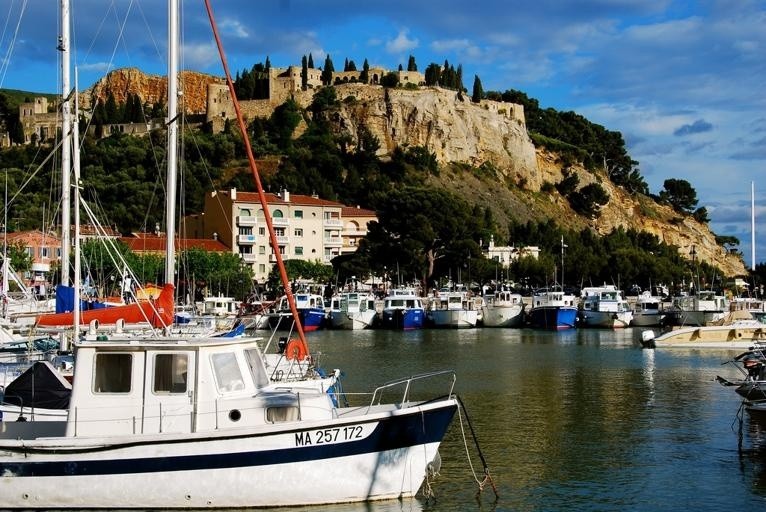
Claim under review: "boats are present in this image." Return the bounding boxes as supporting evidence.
[0,316,465,511]
[1,0,766,415]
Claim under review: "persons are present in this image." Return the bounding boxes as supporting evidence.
[123,270,137,305]
